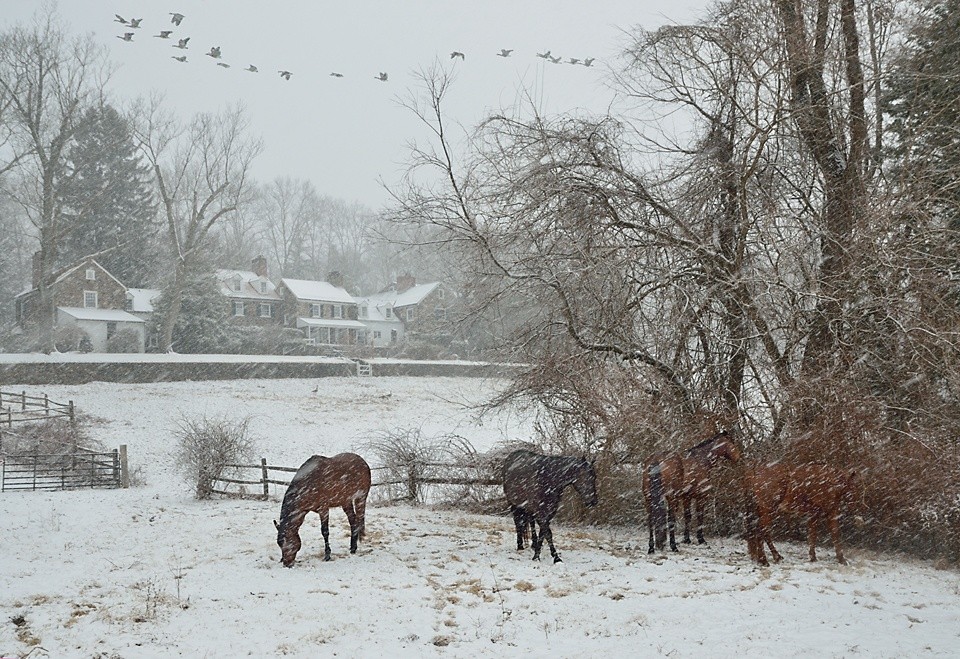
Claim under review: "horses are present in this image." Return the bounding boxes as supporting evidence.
[642,430,857,570]
[503,449,596,563]
[273,452,372,568]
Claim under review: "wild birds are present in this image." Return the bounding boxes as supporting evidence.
[113,9,597,82]
